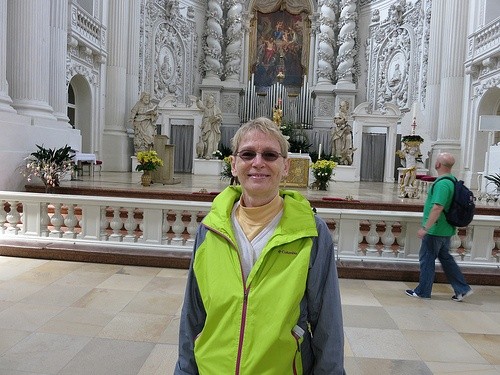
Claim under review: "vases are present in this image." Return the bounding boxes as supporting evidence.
[318,171,332,191]
[229,177,240,186]
[141,171,153,186]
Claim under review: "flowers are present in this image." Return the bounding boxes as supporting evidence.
[135,149,164,173]
[16,158,59,190]
[309,158,338,190]
[217,155,236,180]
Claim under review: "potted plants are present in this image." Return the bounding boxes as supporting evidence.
[401,133,425,146]
[30,143,77,186]
[484,172,500,194]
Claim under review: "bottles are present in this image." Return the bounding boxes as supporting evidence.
[290,324,305,342]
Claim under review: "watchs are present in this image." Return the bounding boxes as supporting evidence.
[423,226,428,231]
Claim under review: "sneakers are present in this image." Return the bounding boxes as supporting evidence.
[406,289,430,299]
[452,289,473,302]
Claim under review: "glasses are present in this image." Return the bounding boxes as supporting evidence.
[236,150,285,161]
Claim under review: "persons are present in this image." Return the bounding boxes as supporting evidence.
[129,91,158,151]
[160,23,169,47]
[196,94,223,160]
[396,29,406,48]
[168,26,177,48]
[389,63,401,86]
[196,136,207,159]
[329,111,352,165]
[402,146,418,188]
[170,0,185,20]
[273,104,283,128]
[404,151,473,302]
[390,5,399,26]
[162,55,172,79]
[383,31,397,56]
[173,117,346,375]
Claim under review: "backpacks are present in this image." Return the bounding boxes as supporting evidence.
[433,177,476,227]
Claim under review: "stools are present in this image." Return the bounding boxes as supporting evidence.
[83,160,103,176]
[400,174,428,192]
[420,176,437,196]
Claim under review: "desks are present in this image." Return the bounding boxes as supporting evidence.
[71,152,97,178]
[279,157,311,188]
[397,167,429,184]
